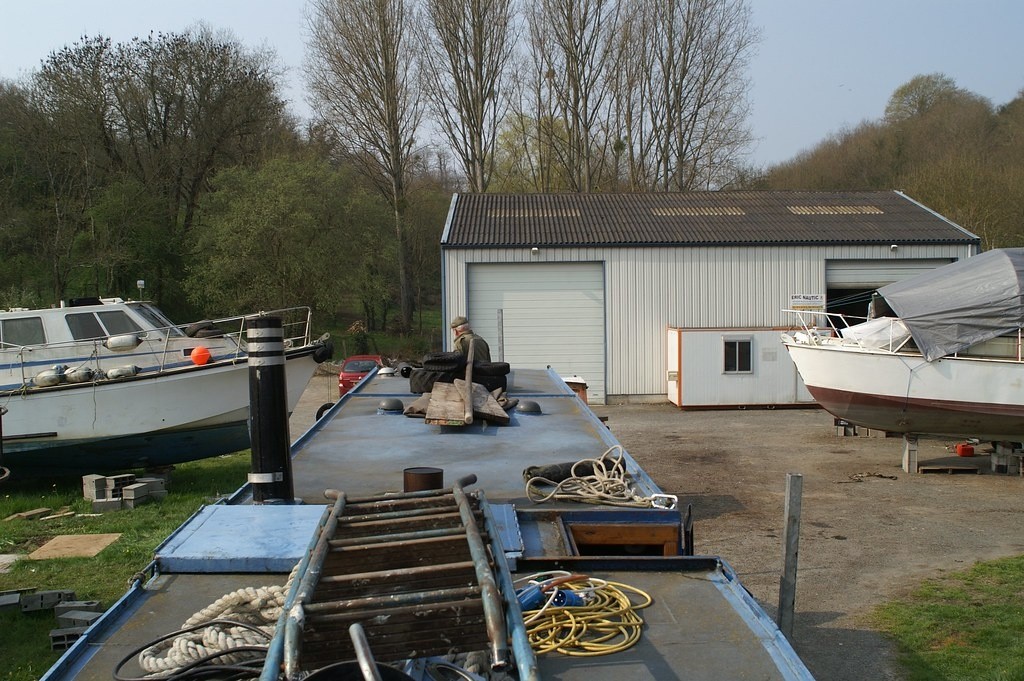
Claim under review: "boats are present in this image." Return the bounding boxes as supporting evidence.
[0,296,330,479]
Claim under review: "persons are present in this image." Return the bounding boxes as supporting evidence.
[450,316,492,363]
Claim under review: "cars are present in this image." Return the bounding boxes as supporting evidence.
[339,354,389,398]
[779,247,1024,447]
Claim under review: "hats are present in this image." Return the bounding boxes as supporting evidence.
[451,316,468,328]
